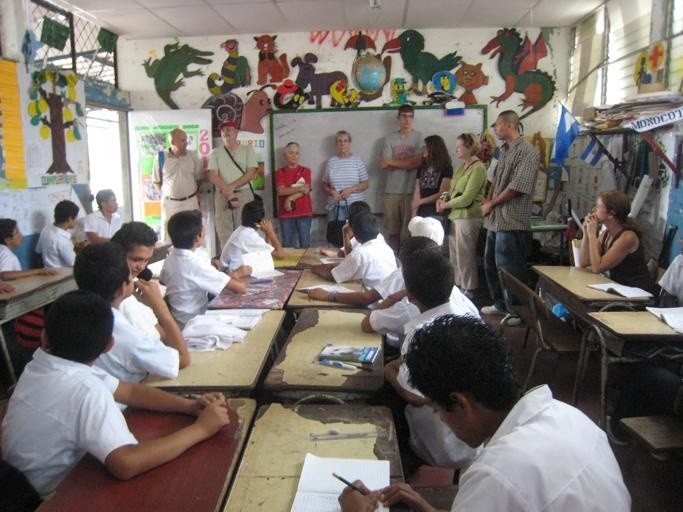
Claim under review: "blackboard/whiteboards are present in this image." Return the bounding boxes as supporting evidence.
[269,105,488,219]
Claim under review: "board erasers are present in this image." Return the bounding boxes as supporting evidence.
[447,108,464,115]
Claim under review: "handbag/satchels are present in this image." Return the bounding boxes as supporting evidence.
[326,197,351,244]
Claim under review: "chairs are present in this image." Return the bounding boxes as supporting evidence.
[498,266,600,397]
[650,265,667,307]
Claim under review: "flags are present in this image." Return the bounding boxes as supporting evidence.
[549,106,580,167]
[580,135,604,167]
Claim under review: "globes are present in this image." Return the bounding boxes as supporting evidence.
[351,56,387,92]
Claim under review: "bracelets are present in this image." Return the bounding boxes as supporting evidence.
[327,290,337,303]
[351,186,356,193]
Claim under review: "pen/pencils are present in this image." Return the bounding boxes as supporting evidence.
[332,472,366,496]
[346,363,372,372]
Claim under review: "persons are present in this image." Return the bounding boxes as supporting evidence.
[0,289,229,511]
[158,210,246,332]
[151,128,202,242]
[578,189,659,310]
[657,232,682,308]
[409,135,452,237]
[310,210,398,308]
[605,362,682,462]
[73,240,190,417]
[308,215,444,356]
[341,204,385,258]
[205,121,259,252]
[375,104,424,239]
[321,130,369,246]
[33,199,91,269]
[0,283,16,296]
[479,109,539,325]
[219,200,284,275]
[380,247,485,470]
[108,220,169,347]
[274,142,312,248]
[317,200,371,257]
[0,218,57,281]
[435,132,487,299]
[337,313,631,512]
[360,236,481,410]
[191,208,251,280]
[83,189,124,244]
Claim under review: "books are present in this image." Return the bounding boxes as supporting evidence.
[587,282,653,299]
[296,283,355,295]
[645,306,683,334]
[205,306,270,330]
[319,344,378,365]
[319,256,344,265]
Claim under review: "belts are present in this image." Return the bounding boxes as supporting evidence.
[166,193,199,202]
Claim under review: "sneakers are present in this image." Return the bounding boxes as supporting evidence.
[482,304,506,315]
[500,311,522,325]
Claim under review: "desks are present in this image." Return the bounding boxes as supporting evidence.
[530,267,651,384]
[527,215,571,266]
[1,266,79,384]
[206,247,368,334]
[572,311,683,431]
[262,306,386,403]
[223,403,410,512]
[147,242,173,266]
[619,413,683,511]
[139,307,286,399]
[35,397,258,511]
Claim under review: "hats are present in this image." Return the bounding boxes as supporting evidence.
[219,119,236,130]
